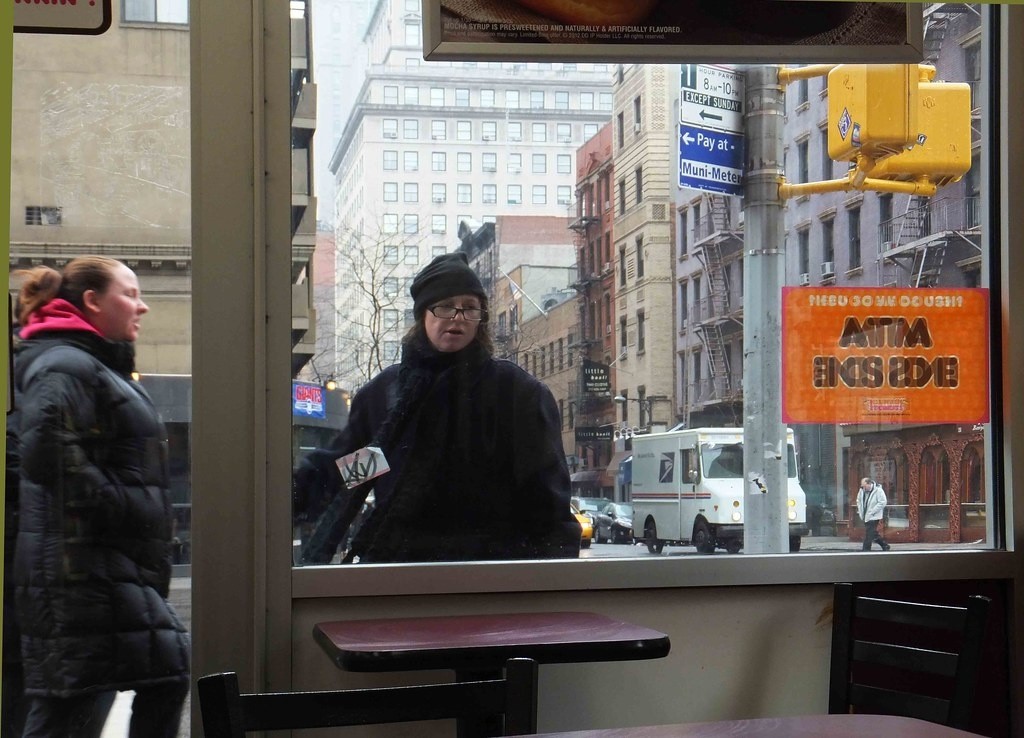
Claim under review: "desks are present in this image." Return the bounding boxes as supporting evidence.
[313,610,671,738]
[493,715,989,738]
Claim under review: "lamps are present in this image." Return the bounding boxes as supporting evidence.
[613,395,674,406]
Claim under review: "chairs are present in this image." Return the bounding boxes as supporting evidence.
[197,656,540,738]
[822,582,991,730]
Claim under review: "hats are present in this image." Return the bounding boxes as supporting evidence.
[409,251,484,319]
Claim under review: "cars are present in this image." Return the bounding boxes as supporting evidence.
[571,494,633,550]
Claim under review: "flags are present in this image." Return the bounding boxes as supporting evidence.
[509,279,524,301]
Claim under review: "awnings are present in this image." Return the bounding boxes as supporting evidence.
[619,455,632,485]
[569,471,599,482]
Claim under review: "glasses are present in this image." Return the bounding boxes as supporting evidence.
[426,305,488,321]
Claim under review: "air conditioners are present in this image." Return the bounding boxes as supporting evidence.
[821,262,834,277]
[881,242,891,253]
[800,272,810,286]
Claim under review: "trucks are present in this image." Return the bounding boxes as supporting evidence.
[632,421,806,555]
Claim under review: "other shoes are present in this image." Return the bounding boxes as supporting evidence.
[883,545,890,551]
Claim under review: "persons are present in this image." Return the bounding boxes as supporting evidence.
[10,256,191,738]
[296,252,582,566]
[856,477,890,552]
